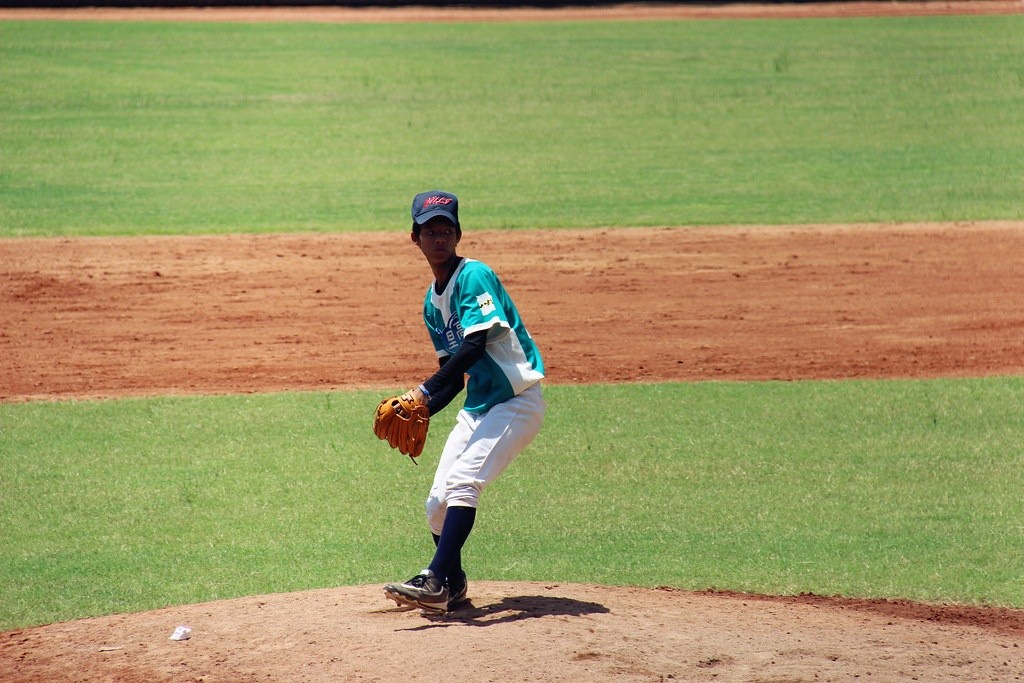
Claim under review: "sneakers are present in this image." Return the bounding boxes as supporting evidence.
[445,572,466,606]
[382,567,449,618]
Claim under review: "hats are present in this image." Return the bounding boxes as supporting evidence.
[411,191,458,225]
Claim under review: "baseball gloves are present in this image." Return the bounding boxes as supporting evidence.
[372,388,430,458]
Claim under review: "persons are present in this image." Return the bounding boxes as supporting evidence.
[372,191,544,616]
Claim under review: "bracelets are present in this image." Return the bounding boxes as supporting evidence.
[419,384,429,396]
